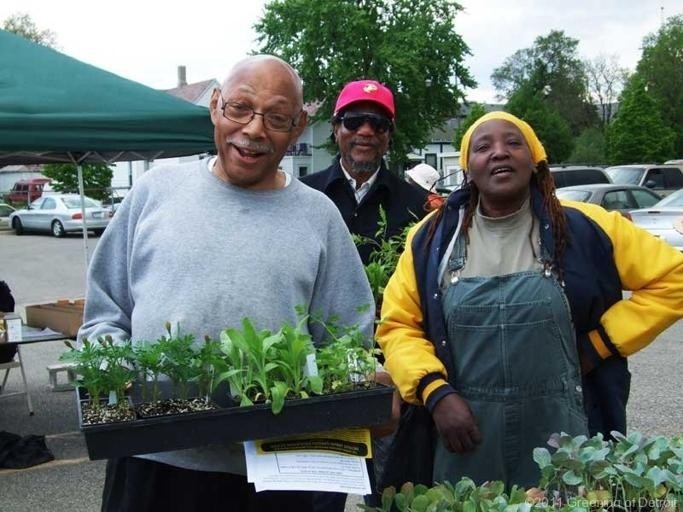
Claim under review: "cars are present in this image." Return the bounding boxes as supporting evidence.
[430,158,683,255]
[0,177,133,238]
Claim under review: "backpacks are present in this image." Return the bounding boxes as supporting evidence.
[422,192,445,212]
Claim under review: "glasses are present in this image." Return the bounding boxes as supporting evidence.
[216,87,304,134]
[336,110,393,134]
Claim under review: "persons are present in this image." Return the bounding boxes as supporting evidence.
[404,163,445,209]
[77,54,376,512]
[374,113,683,497]
[296,80,446,511]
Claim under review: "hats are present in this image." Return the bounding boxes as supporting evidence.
[332,79,396,120]
[403,162,440,194]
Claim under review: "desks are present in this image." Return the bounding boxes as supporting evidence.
[0,324,69,346]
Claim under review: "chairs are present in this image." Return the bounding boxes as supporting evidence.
[0,344,34,415]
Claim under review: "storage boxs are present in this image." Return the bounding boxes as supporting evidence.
[25,299,84,338]
